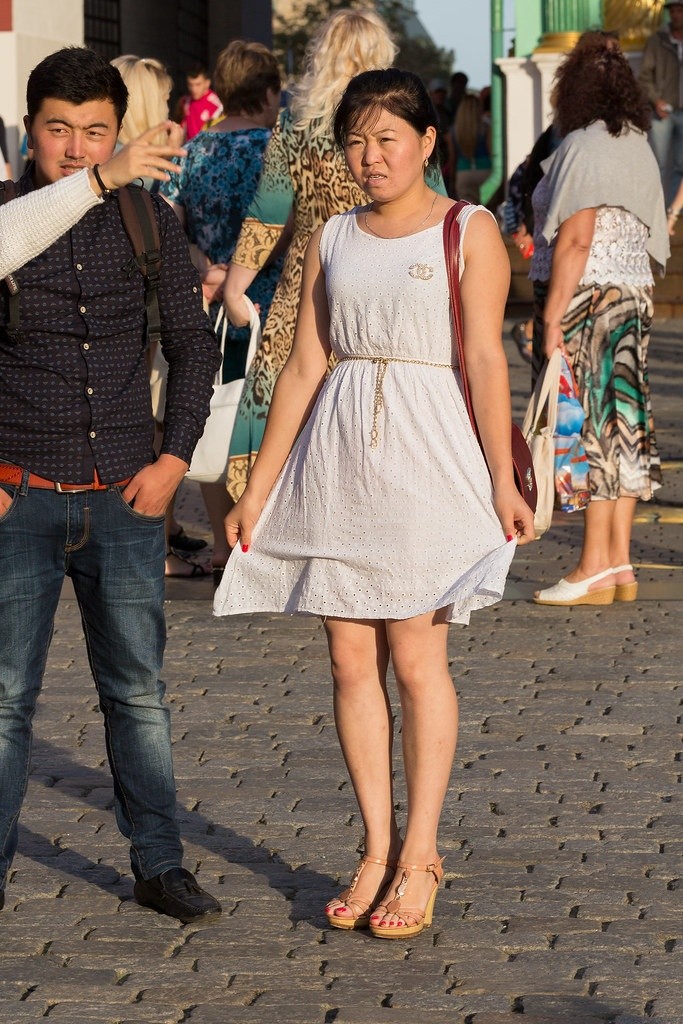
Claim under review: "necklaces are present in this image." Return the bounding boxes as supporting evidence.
[365,195,438,240]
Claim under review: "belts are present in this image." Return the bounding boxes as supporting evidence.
[0,463,153,494]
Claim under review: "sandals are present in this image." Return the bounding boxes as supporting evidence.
[164,550,211,577]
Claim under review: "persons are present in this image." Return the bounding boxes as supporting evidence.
[508,0,683,606]
[1,12,448,924]
[214,69,537,938]
[429,72,491,202]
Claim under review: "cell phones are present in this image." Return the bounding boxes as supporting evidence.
[512,233,534,258]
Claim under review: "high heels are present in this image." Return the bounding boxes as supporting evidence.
[211,548,234,585]
[527,569,615,607]
[371,851,447,940]
[326,840,404,930]
[613,563,638,602]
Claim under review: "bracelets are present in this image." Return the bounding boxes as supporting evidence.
[667,214,678,221]
[667,208,680,216]
[93,164,111,195]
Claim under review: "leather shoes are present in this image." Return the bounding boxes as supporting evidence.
[132,868,222,921]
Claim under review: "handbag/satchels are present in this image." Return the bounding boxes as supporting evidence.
[518,349,565,540]
[511,420,538,517]
[183,293,262,484]
[555,357,590,514]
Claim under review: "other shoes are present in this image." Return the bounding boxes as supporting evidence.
[168,528,208,552]
